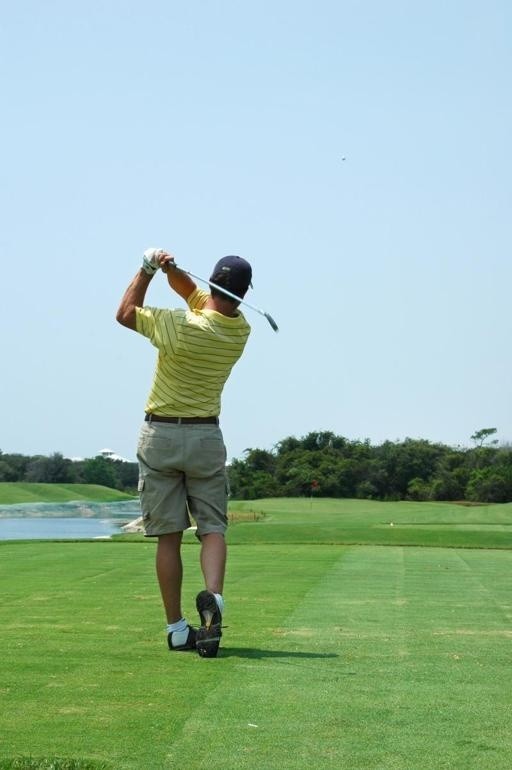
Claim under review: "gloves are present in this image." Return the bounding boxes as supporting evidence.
[140,247,168,275]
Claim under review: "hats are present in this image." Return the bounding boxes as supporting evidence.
[211,254,253,291]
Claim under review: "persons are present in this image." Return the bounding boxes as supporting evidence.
[114,246,257,660]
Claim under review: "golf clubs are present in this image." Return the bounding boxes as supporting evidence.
[166,262,279,333]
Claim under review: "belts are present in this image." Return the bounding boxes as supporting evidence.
[143,414,220,425]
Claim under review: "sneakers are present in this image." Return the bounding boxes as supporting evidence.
[166,624,198,651]
[194,591,225,660]
